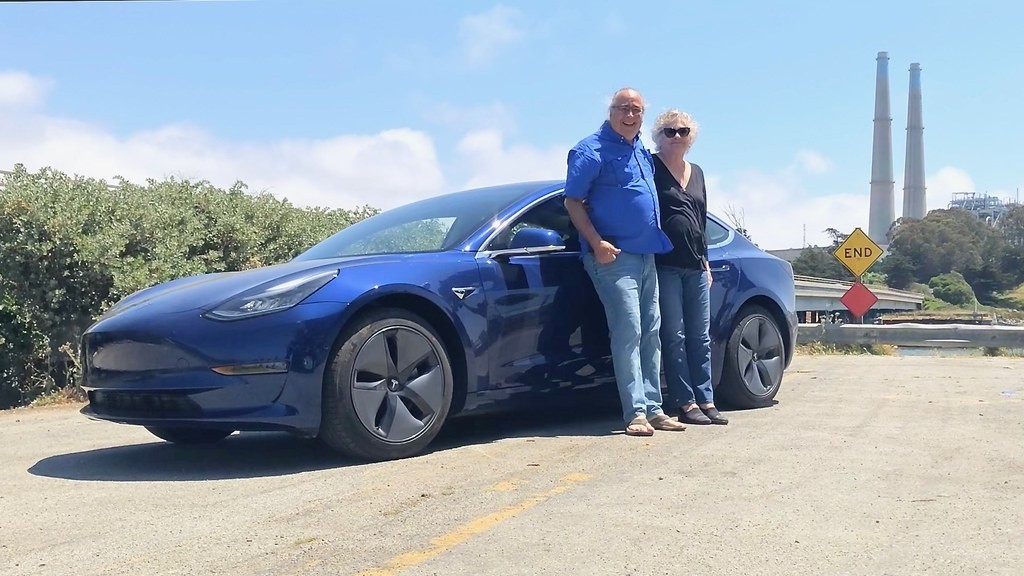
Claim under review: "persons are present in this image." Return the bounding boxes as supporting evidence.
[563,87,688,438]
[647,108,729,427]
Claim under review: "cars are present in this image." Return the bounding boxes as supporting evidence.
[79,180,798,461]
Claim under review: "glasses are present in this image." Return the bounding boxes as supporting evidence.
[660,128,690,138]
[612,106,643,115]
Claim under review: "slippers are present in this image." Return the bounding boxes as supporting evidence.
[626,415,686,435]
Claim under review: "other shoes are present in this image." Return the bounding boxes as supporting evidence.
[678,404,728,425]
